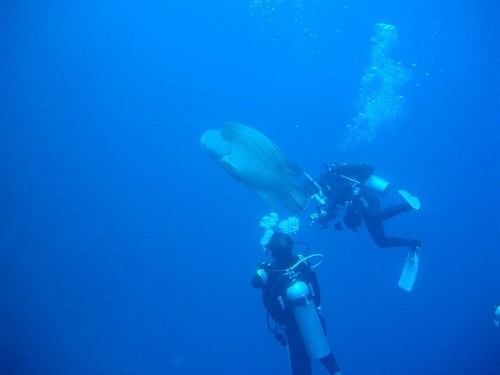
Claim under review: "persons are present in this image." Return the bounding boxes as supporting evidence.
[304,162,422,292]
[262,232,340,375]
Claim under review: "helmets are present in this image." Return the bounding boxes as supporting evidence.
[270,232,293,261]
[304,177,317,192]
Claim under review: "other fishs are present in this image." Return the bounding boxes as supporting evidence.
[199,122,321,214]
